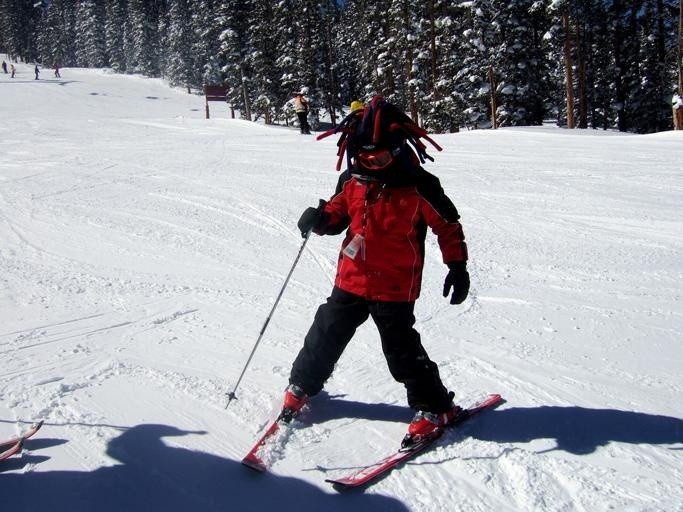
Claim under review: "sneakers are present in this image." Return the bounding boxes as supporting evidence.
[284,384,309,410]
[408,404,456,437]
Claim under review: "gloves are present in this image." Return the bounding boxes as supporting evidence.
[297,207,320,234]
[443,262,470,304]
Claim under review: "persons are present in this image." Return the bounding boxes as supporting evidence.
[295,86,312,135]
[11,65,16,77]
[34,64,40,79]
[54,64,61,78]
[2,60,8,74]
[284,96,470,436]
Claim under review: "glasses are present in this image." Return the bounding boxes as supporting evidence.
[357,148,400,170]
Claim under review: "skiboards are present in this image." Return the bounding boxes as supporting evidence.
[239,393,502,486]
[0,420,43,460]
